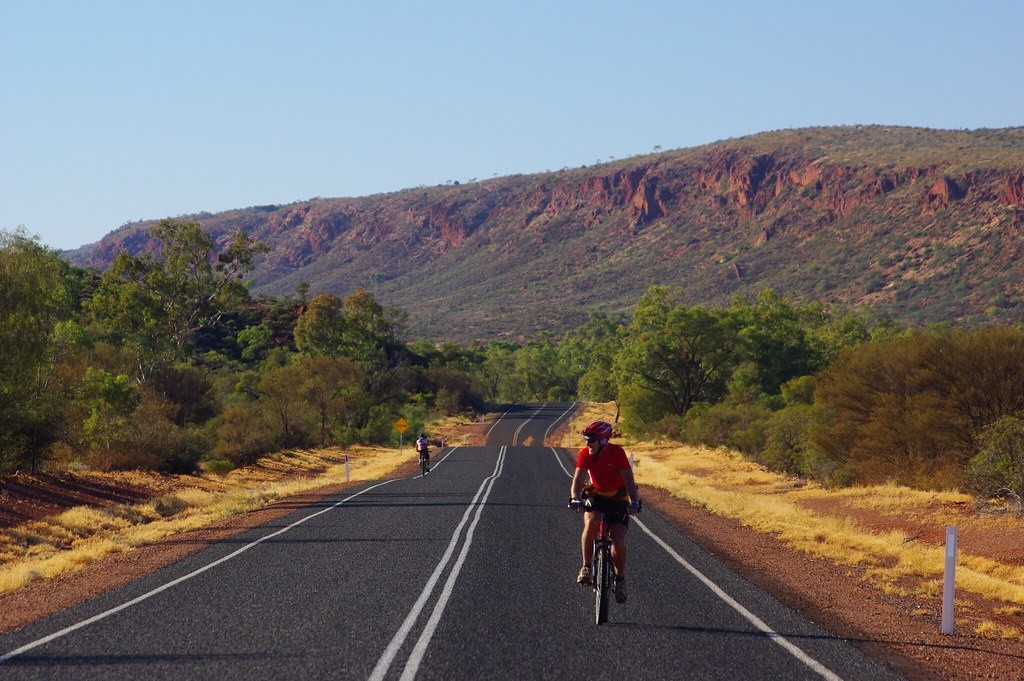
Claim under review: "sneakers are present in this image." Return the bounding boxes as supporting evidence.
[576,566,592,583]
[613,576,628,603]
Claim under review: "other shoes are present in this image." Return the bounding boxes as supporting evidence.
[426,468,430,472]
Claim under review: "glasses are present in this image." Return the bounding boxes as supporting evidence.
[585,438,599,443]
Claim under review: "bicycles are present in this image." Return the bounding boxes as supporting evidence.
[419,450,432,477]
[568,498,642,625]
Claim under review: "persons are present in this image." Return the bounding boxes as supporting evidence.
[568,421,639,603]
[415,433,430,472]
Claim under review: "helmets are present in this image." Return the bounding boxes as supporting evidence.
[419,434,427,438]
[581,422,613,438]
